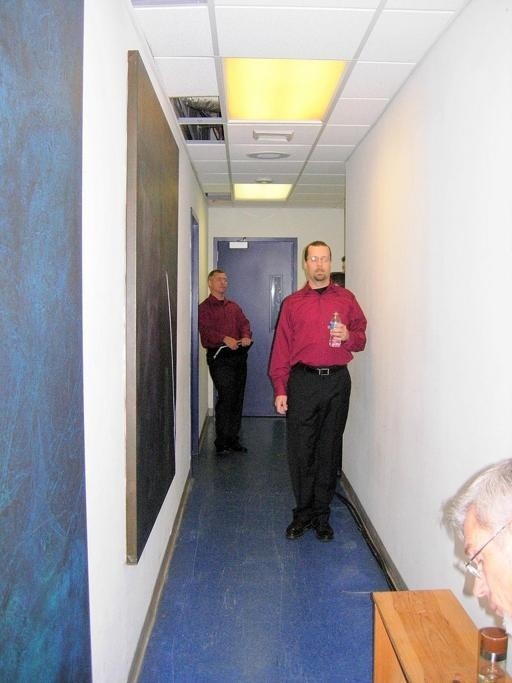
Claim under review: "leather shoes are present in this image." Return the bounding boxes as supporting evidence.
[213,436,248,456]
[286,515,333,541]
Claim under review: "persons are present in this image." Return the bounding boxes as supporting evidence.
[268,241,368,541]
[449,458,512,619]
[198,270,251,454]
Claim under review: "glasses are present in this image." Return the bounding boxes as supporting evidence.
[465,522,509,578]
[306,256,332,263]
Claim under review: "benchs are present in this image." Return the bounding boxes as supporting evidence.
[368,586,508,683]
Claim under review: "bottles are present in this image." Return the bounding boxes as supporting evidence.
[476,627,509,682]
[328,311,342,348]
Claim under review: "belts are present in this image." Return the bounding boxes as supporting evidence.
[297,360,346,376]
